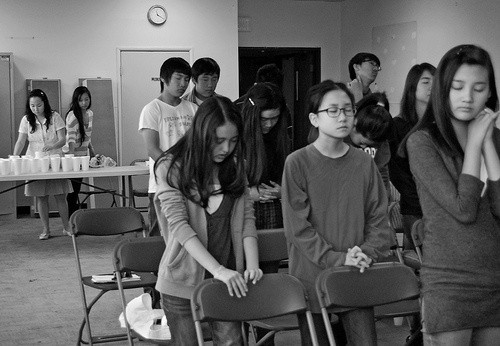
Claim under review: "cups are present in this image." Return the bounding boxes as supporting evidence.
[81,156,90,170]
[0,152,50,176]
[50,154,60,172]
[62,154,81,171]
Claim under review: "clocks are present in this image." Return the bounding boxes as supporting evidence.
[147,5,168,25]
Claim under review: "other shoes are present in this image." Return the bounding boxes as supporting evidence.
[63,229,72,236]
[39,233,50,240]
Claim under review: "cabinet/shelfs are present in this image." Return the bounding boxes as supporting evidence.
[0,52,17,222]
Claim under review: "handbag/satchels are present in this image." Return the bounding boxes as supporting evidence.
[119,293,171,340]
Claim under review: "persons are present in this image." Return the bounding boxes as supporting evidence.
[233,56,294,346]
[65,86,96,222]
[138,56,200,326]
[12,81,74,240]
[182,58,220,106]
[346,52,382,100]
[392,63,437,346]
[153,96,266,346]
[348,90,392,207]
[408,45,500,346]
[282,79,392,344]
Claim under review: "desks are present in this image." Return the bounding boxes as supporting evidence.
[0,166,150,238]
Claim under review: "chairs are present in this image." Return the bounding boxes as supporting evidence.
[112,236,213,346]
[69,207,157,346]
[412,220,425,264]
[77,154,125,237]
[128,159,150,237]
[244,229,300,346]
[388,201,423,264]
[191,273,318,346]
[315,262,421,346]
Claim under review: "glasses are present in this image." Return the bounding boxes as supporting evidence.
[315,107,357,118]
[364,61,381,70]
